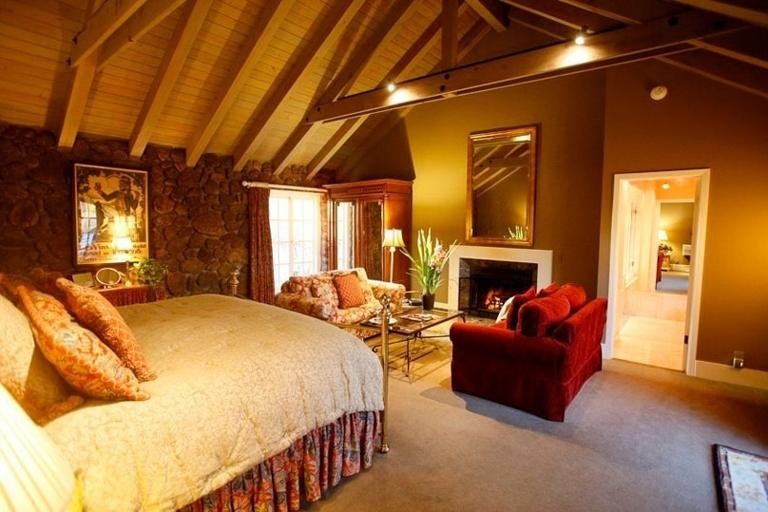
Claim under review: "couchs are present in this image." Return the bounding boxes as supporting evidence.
[274,266,406,342]
[451,281,608,422]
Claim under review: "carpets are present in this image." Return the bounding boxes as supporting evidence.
[714,443,768,511]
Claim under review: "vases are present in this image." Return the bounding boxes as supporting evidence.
[420,294,435,311]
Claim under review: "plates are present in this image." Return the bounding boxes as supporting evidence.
[369,316,397,325]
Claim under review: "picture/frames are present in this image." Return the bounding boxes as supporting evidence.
[68,159,153,271]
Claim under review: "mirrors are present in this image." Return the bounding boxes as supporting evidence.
[465,125,537,248]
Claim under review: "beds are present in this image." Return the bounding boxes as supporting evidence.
[0,293,392,512]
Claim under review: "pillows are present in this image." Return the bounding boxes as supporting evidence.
[285,271,368,308]
[0,265,159,426]
[494,282,560,330]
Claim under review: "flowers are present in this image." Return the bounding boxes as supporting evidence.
[399,225,463,296]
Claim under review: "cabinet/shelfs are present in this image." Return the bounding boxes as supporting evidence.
[321,178,413,300]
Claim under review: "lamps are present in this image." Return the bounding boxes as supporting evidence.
[381,227,405,282]
